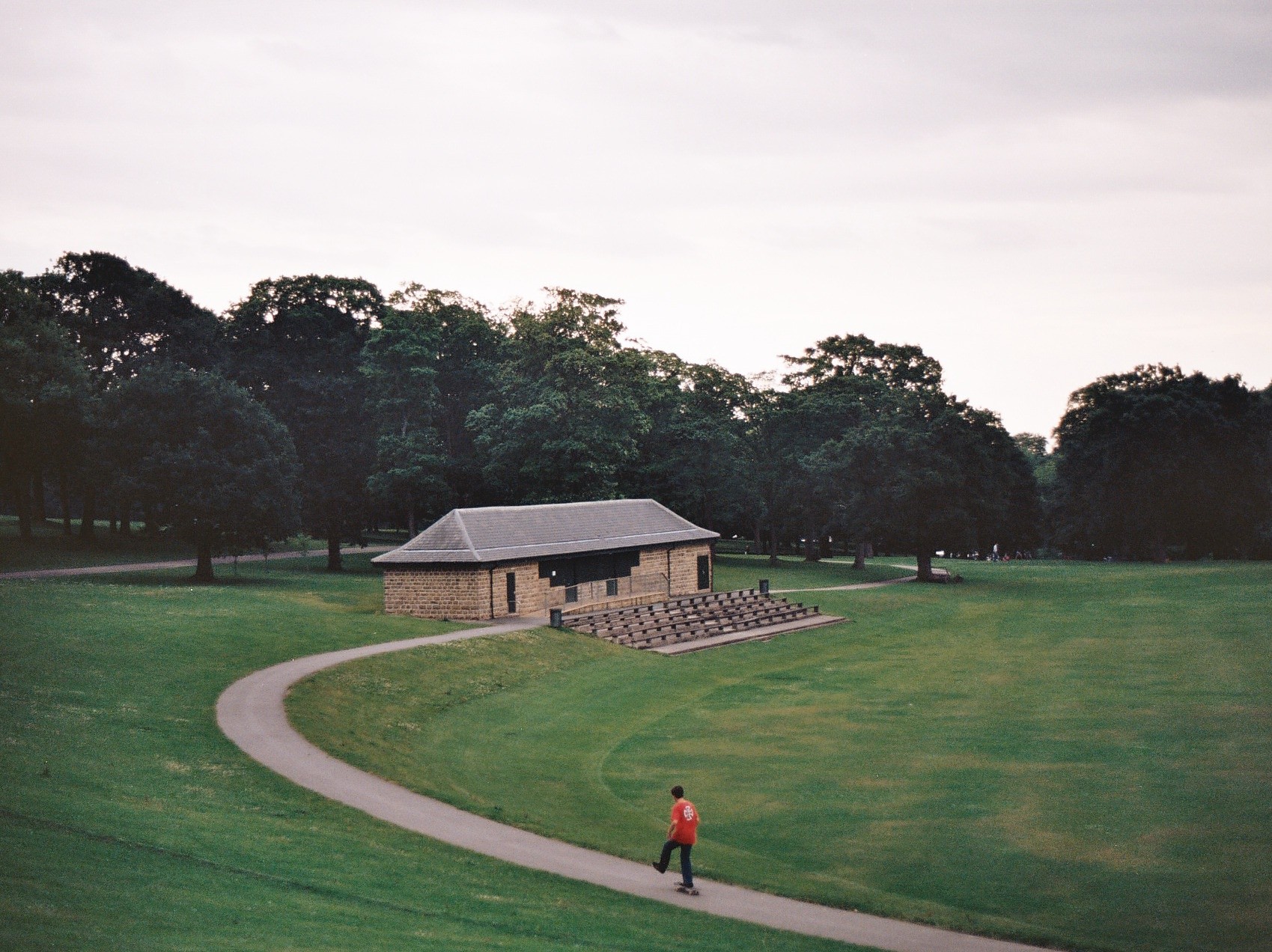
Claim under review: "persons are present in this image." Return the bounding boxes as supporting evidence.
[652,785,700,888]
[993,542,999,562]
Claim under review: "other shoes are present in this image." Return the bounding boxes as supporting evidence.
[652,862,663,874]
[680,882,693,889]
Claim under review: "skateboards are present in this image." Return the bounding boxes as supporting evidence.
[673,880,702,896]
